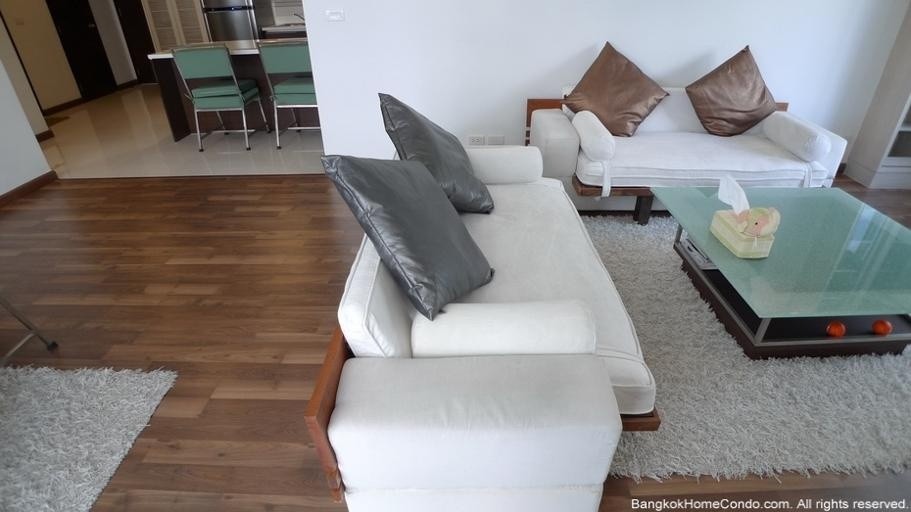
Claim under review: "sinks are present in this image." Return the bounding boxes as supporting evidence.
[275,23,305,27]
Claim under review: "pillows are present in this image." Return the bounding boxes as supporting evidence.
[561,43,779,138]
[321,93,496,320]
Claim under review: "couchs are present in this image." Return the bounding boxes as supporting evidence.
[304,145,663,512]
[531,87,848,224]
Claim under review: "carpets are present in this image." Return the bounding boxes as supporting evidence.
[0,361,180,511]
[581,215,911,482]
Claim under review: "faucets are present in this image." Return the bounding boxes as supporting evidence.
[294,14,304,21]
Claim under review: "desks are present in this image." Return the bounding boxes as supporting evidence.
[146,38,319,142]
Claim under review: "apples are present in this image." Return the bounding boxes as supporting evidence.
[872,319,892,335]
[826,321,846,337]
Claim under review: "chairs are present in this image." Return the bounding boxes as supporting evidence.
[171,37,321,151]
